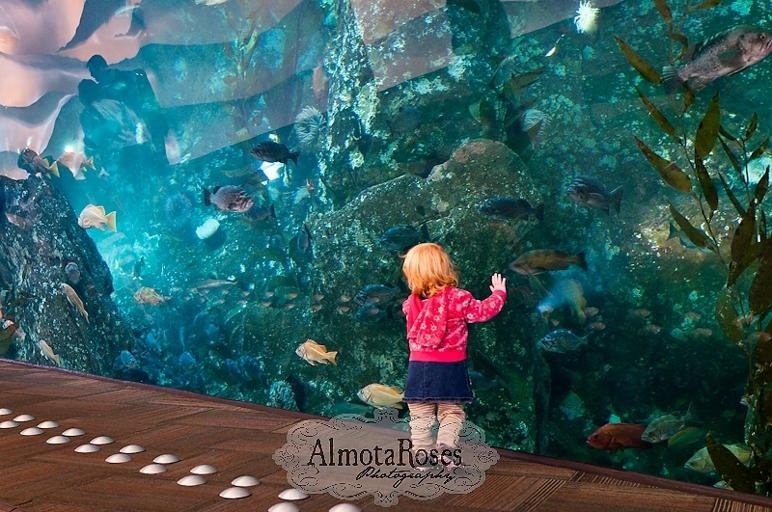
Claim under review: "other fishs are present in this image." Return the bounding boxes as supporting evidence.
[585,407,768,497]
[534,327,596,359]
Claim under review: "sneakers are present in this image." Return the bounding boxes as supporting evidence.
[413,443,465,472]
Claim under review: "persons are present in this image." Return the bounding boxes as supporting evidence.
[396,242,507,472]
